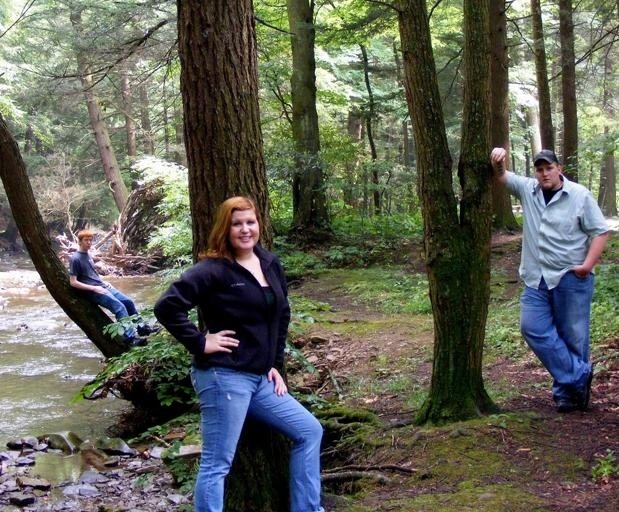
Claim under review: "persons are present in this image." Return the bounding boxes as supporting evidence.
[153,195,327,511]
[488,143,612,417]
[68,228,155,346]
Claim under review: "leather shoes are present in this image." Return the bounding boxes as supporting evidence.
[556,400,579,413]
[579,373,594,409]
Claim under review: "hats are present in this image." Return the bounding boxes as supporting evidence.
[533,149,555,167]
